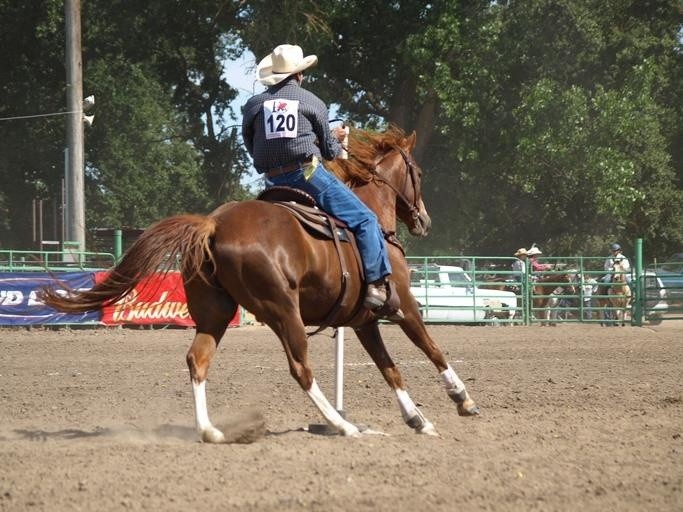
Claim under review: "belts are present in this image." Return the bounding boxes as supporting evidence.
[267,159,312,176]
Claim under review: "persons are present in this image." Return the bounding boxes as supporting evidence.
[241,43,405,321]
[512,242,630,323]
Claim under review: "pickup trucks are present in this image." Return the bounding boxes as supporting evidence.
[408,264,518,326]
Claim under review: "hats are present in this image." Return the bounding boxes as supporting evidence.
[515,248,542,256]
[255,43,319,85]
[609,243,620,251]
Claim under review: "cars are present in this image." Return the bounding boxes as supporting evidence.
[646,252,683,313]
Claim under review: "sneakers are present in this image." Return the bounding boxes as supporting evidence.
[364,284,405,321]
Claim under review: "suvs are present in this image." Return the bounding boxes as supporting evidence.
[626,271,669,324]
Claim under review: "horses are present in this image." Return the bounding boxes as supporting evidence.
[596,273,632,326]
[477,268,586,327]
[26,120,480,444]
